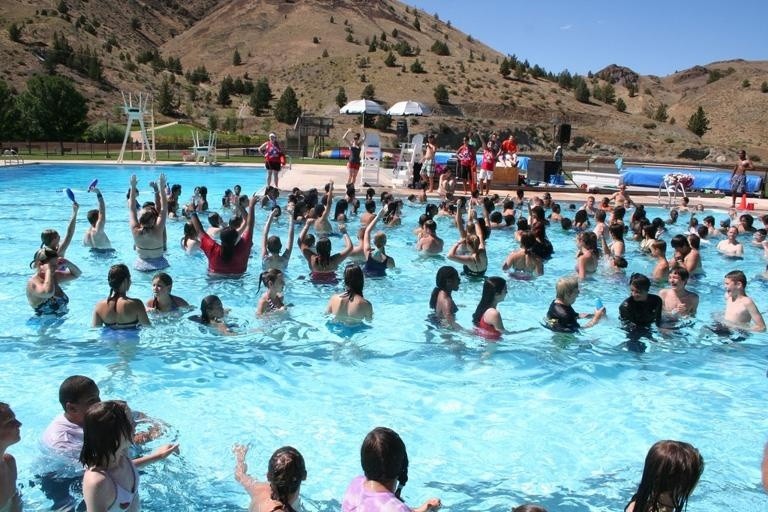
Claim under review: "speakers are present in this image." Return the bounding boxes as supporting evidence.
[396,119,408,139]
[557,124,571,144]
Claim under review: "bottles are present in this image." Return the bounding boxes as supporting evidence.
[594,297,608,322]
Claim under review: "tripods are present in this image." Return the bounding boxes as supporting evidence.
[546,144,580,189]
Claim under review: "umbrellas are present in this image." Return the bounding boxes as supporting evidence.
[386,100,433,148]
[339,98,387,149]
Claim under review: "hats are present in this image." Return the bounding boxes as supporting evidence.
[268,133,276,138]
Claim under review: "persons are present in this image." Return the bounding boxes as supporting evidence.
[730,150,754,209]
[25,168,768,335]
[514,440,705,512]
[43,375,180,512]
[1,401,23,506]
[408,133,563,195]
[237,427,441,512]
[259,134,286,191]
[343,129,366,186]
